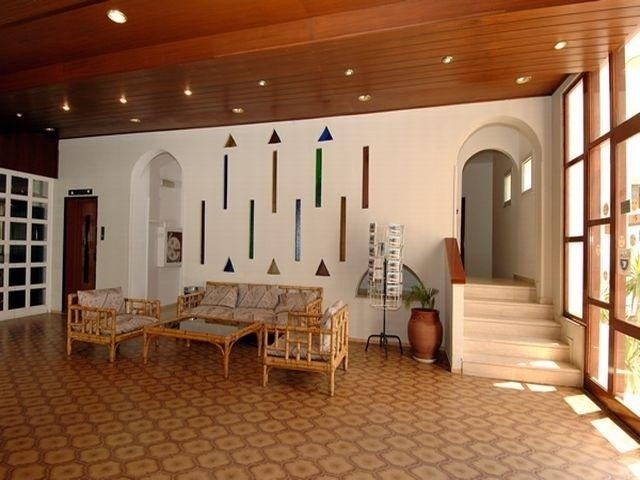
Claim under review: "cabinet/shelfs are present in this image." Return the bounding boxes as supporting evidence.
[359,220,406,360]
[0,167,56,321]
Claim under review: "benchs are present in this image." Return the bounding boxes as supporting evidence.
[174,280,324,346]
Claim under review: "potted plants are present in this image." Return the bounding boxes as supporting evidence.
[401,283,445,369]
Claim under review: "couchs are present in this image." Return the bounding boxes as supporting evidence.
[65,286,160,365]
[261,299,352,396]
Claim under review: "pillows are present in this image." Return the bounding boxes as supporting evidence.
[77,286,125,324]
[318,300,345,359]
[198,283,322,313]
